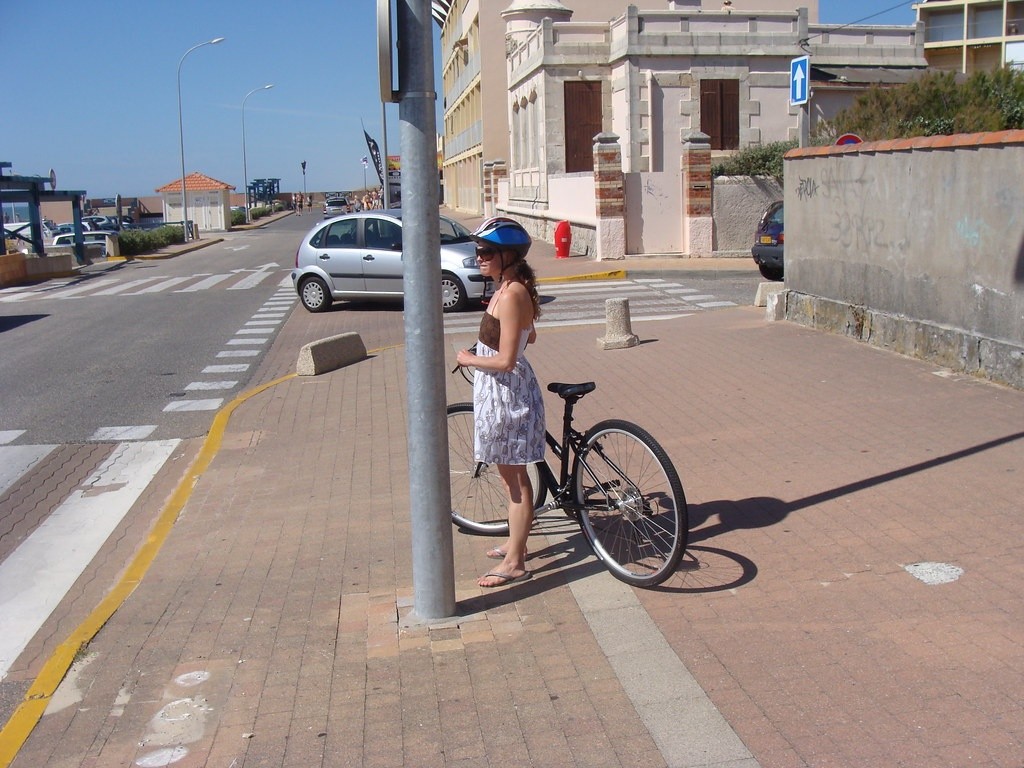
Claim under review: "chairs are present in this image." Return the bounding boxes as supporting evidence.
[363,230,389,248]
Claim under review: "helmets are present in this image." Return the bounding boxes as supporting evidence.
[469,217,532,258]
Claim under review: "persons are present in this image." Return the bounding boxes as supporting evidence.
[354,188,383,212]
[292,192,297,209]
[306,195,312,214]
[457,217,546,587]
[296,191,304,216]
[3,212,9,223]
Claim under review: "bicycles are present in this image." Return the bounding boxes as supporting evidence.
[444,339,691,588]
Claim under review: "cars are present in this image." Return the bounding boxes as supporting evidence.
[751,198,784,279]
[291,209,497,313]
[322,197,353,217]
[230,206,245,214]
[1,213,134,261]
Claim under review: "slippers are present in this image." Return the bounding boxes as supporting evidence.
[487,546,527,561]
[478,569,532,587]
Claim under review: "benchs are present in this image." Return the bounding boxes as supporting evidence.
[328,234,355,245]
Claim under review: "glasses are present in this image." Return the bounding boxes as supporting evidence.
[476,246,501,261]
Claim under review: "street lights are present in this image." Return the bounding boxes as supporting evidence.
[241,84,275,224]
[177,36,226,244]
